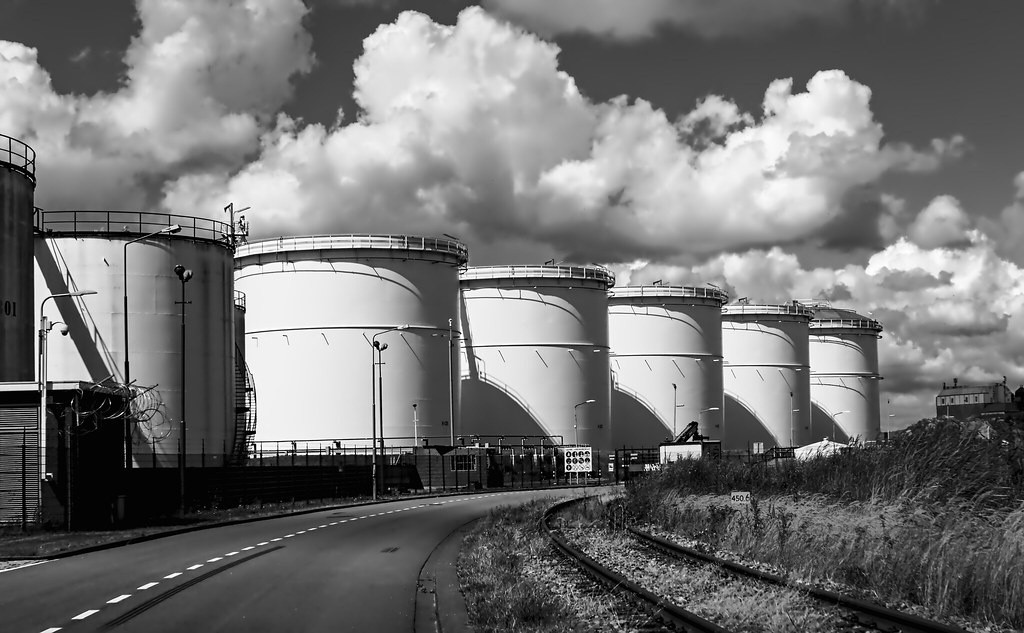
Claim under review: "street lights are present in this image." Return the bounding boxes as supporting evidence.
[374,340,388,498]
[37,289,99,527]
[888,415,896,441]
[574,400,596,485]
[700,407,720,435]
[833,411,851,457]
[372,323,409,501]
[172,263,194,512]
[123,223,183,465]
